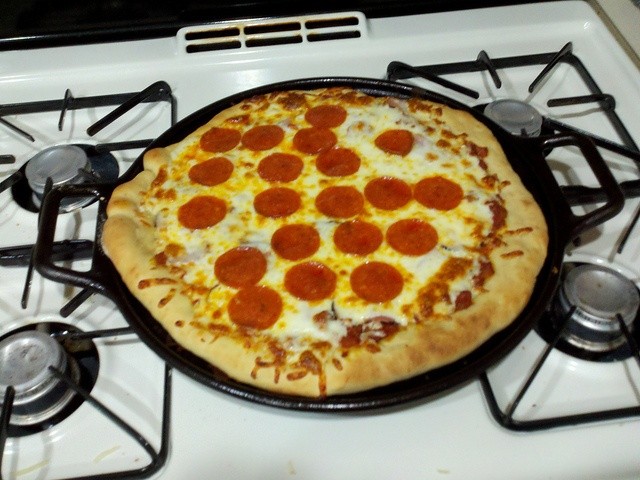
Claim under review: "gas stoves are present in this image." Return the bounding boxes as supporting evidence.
[0,0,640,478]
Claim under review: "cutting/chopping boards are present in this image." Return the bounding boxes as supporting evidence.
[35,76,625,414]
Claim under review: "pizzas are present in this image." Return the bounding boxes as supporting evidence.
[99,86,551,399]
